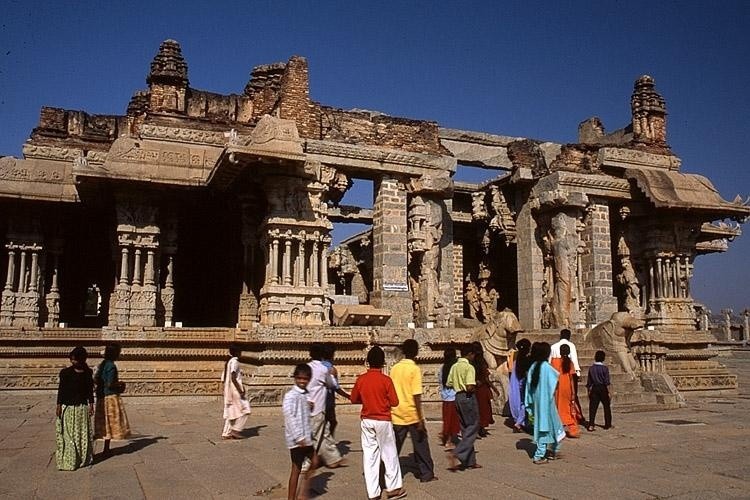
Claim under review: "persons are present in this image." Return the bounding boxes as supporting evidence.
[524,341,567,465]
[220,344,251,440]
[548,329,587,425]
[300,344,354,475]
[54,347,96,473]
[469,341,500,439]
[584,349,614,431]
[377,339,440,491]
[508,338,532,434]
[503,341,517,428]
[350,345,408,500]
[282,364,319,500]
[445,344,483,471]
[550,344,583,438]
[321,341,338,436]
[438,342,459,453]
[94,342,133,458]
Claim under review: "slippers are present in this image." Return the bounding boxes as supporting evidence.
[388,489,407,499]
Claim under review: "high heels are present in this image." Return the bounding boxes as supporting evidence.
[513,424,524,433]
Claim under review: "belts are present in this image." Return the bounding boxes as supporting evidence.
[456,390,467,393]
